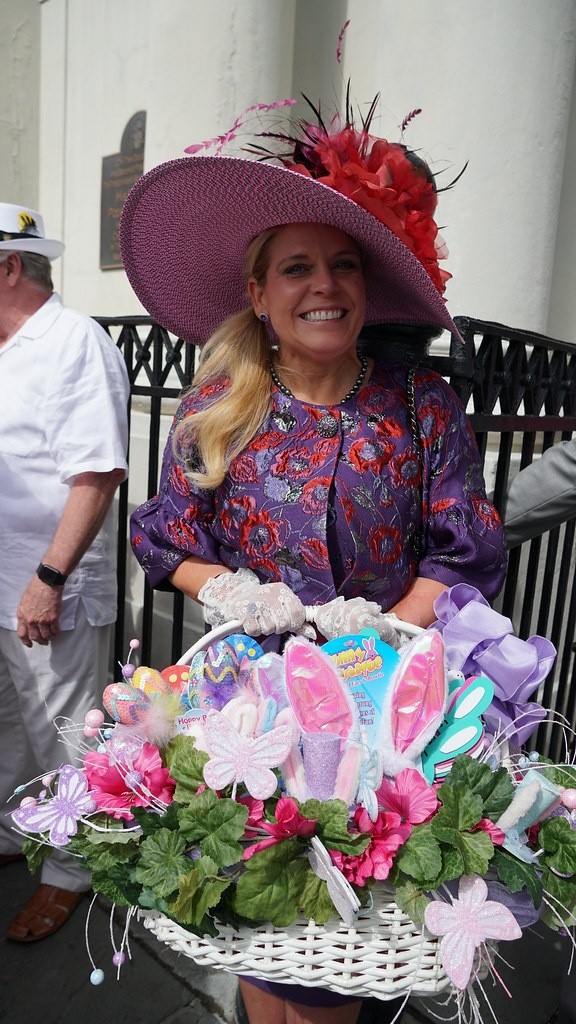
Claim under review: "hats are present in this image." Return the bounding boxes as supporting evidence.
[121,19,469,357]
[0,202,66,261]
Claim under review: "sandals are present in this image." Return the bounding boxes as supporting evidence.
[8,884,88,942]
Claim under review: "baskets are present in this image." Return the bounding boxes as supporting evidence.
[137,603,500,1003]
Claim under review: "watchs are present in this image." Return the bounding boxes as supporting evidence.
[36,562,68,586]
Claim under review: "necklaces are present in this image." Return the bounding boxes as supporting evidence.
[267,347,367,403]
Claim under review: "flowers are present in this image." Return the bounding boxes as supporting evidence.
[181,20,468,304]
[2,584,576,1024]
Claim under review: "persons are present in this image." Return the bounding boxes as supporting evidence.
[0,202,132,943]
[486,437,576,550]
[120,156,507,1024]
